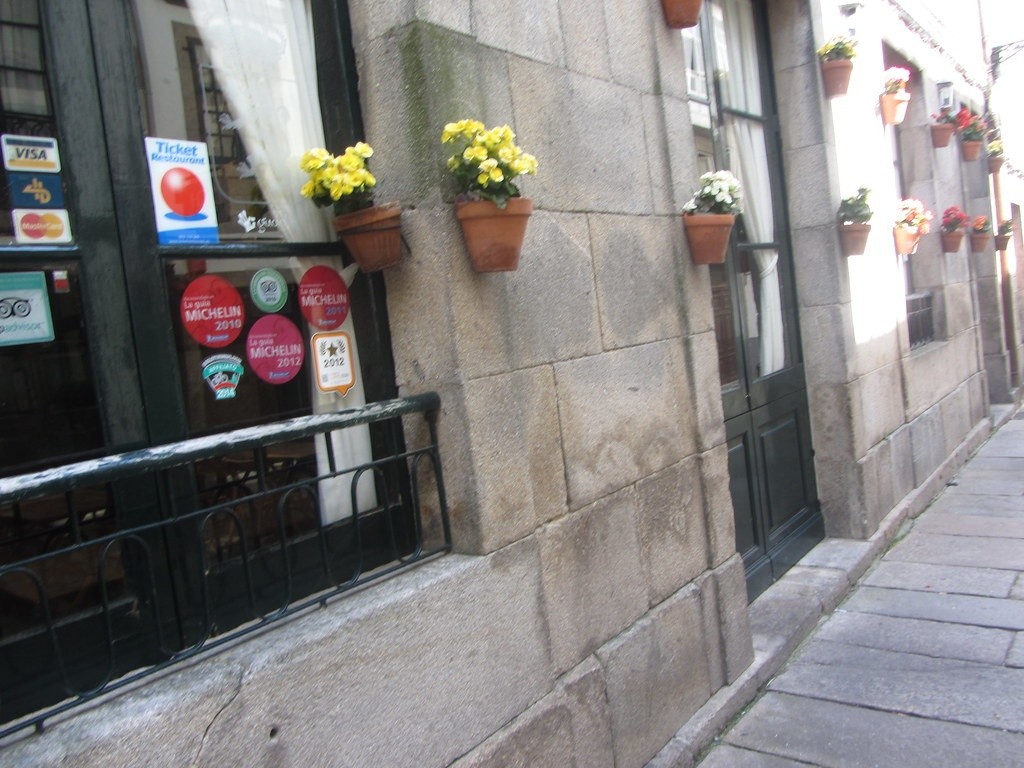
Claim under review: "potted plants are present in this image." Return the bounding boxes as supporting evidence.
[836,188,873,256]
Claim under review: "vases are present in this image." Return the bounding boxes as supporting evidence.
[931,123,954,148]
[662,0,702,29]
[332,201,402,274]
[970,233,990,252]
[994,235,1010,250]
[962,141,983,161]
[941,231,964,252]
[893,226,919,255]
[455,198,532,273]
[821,61,853,99]
[987,156,1004,174]
[880,93,911,126]
[683,213,736,265]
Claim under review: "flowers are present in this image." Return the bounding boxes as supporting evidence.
[681,168,744,217]
[996,217,1016,237]
[815,35,858,65]
[877,67,910,95]
[928,106,1004,158]
[300,141,375,217]
[940,206,971,233]
[441,119,539,210]
[893,198,932,235]
[972,214,993,234]
